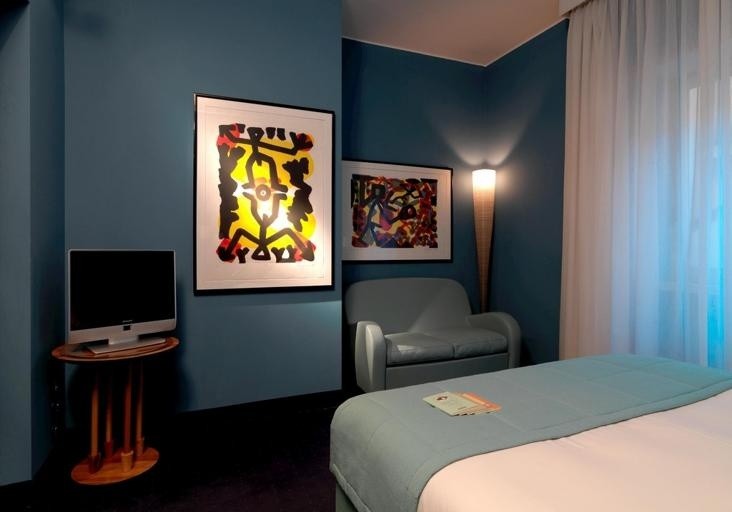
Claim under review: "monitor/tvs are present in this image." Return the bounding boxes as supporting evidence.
[65,248,177,355]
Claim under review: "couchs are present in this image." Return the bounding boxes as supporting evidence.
[344,277,523,392]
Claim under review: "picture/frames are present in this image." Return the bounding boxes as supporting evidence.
[192,91,336,297]
[341,157,453,265]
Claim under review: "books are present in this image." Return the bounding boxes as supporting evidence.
[456,392,503,417]
[420,389,483,417]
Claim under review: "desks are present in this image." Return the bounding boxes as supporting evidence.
[50,335,179,487]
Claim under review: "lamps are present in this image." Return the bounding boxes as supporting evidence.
[472,169,497,313]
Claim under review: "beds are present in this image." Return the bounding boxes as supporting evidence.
[328,352,732,512]
